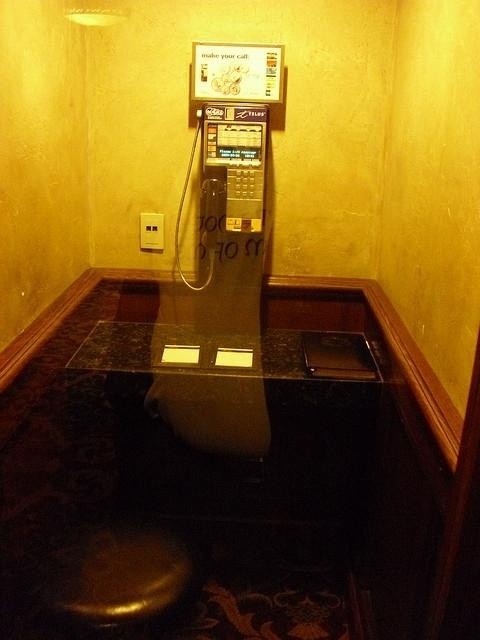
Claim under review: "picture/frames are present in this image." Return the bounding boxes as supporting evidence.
[191,42,285,104]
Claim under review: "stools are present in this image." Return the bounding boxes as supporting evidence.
[44,519,194,635]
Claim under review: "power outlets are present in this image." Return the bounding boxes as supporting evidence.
[140,213,163,252]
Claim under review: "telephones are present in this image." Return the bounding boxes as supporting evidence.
[174,103,269,294]
